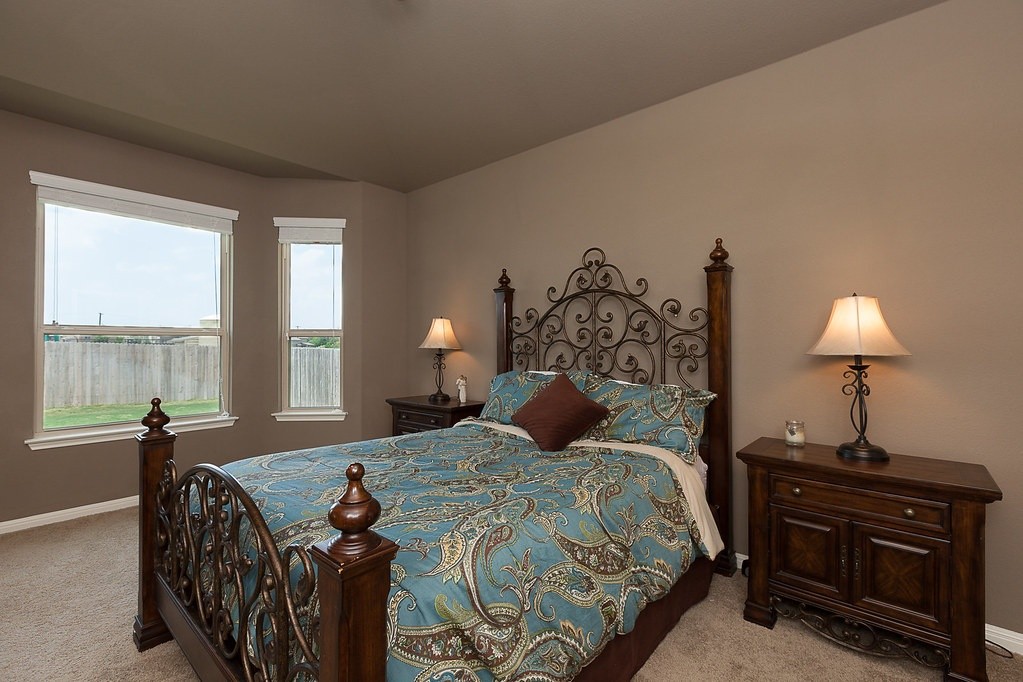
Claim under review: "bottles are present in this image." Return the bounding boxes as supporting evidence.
[785,420,806,447]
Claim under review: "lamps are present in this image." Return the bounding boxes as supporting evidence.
[417,317,463,402]
[804,293,912,461]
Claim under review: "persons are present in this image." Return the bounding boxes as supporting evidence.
[456,375,467,402]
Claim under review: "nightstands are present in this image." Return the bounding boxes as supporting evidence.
[735,436,1003,682]
[385,395,485,436]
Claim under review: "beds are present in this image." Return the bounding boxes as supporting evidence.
[130,238,737,682]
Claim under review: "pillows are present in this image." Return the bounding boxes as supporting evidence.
[581,374,718,465]
[476,371,556,424]
[511,373,609,452]
